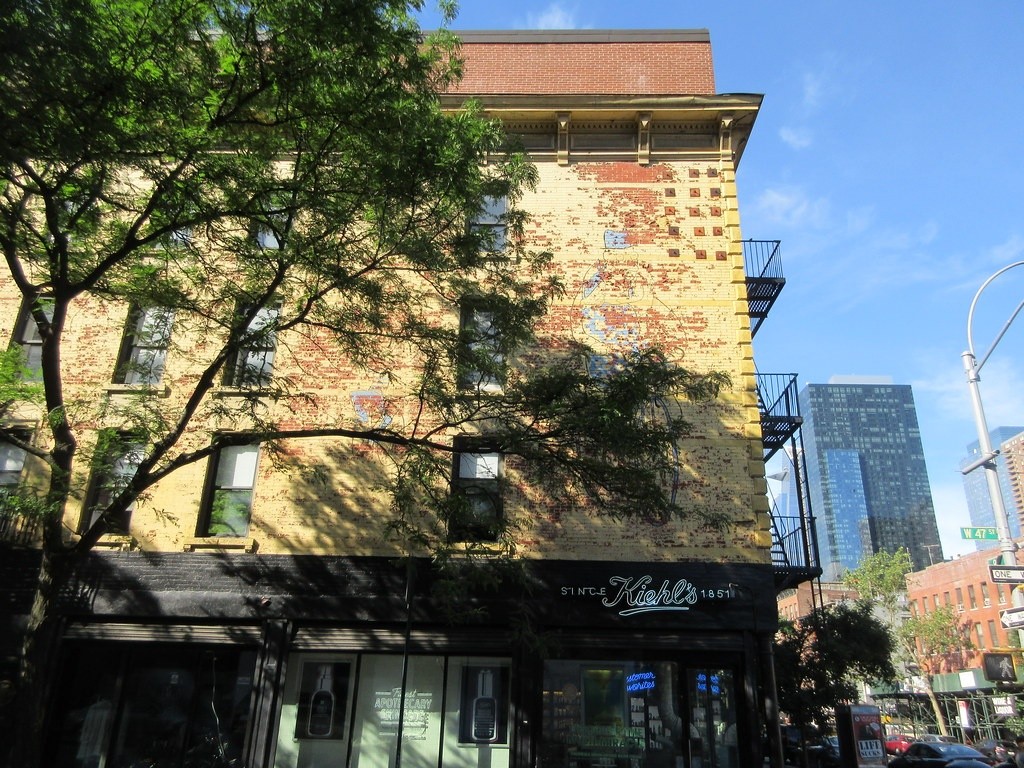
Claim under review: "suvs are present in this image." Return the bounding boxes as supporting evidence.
[969,739,1018,760]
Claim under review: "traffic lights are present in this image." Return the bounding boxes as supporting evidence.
[982,653,1017,683]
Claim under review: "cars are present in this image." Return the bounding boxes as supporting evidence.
[886,742,991,768]
[884,734,917,755]
[915,735,960,743]
[765,724,839,768]
[825,736,839,755]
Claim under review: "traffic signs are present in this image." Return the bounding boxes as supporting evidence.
[998,606,1024,629]
[959,526,999,541]
[988,554,1024,585]
